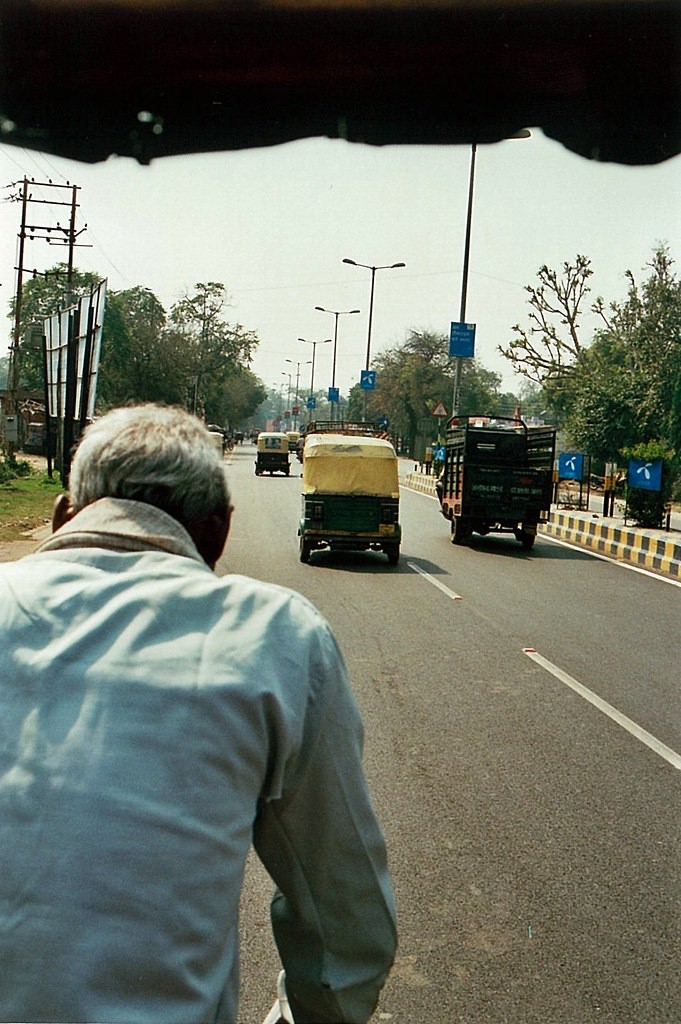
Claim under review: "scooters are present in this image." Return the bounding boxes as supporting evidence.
[255,432,291,476]
[286,432,300,451]
[436,414,557,550]
[298,420,402,566]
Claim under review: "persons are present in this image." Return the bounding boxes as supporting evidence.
[0,403,397,1024]
[432,442,444,479]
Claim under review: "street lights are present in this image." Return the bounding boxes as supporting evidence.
[274,383,289,412]
[315,307,360,422]
[286,359,312,432]
[298,338,332,421]
[452,130,531,429]
[282,373,301,427]
[342,259,406,422]
